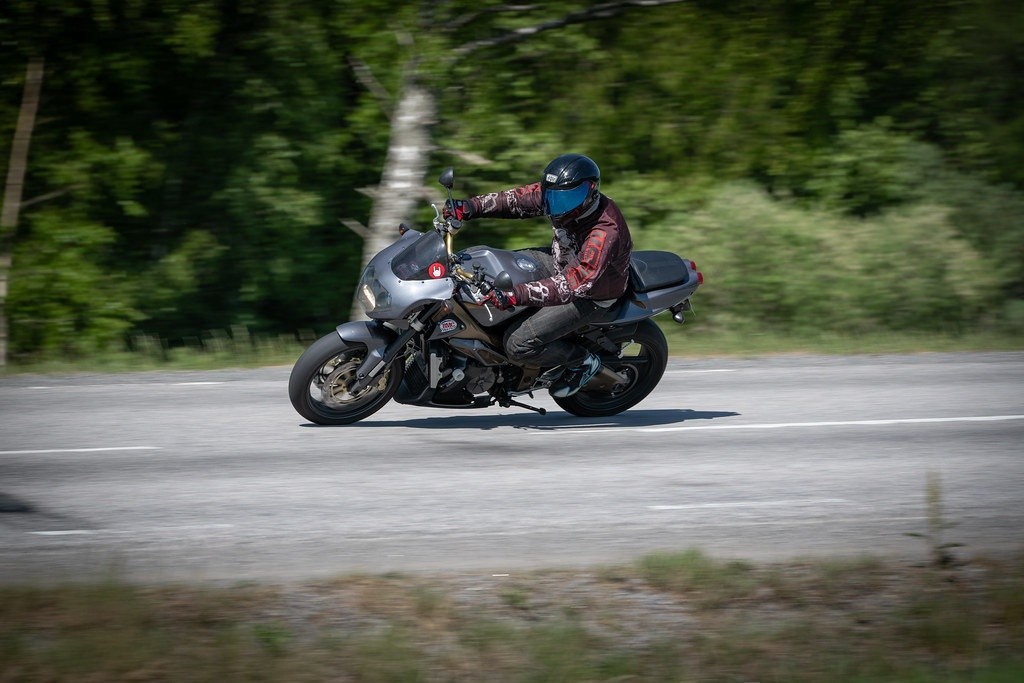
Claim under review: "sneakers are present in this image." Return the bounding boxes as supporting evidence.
[549,353,601,398]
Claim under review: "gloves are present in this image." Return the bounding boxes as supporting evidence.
[442,198,475,221]
[478,288,518,311]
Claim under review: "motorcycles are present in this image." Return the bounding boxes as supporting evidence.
[288,166,704,426]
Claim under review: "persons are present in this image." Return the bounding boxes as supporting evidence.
[442,154,632,397]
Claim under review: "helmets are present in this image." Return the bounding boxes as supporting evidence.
[541,154,601,229]
